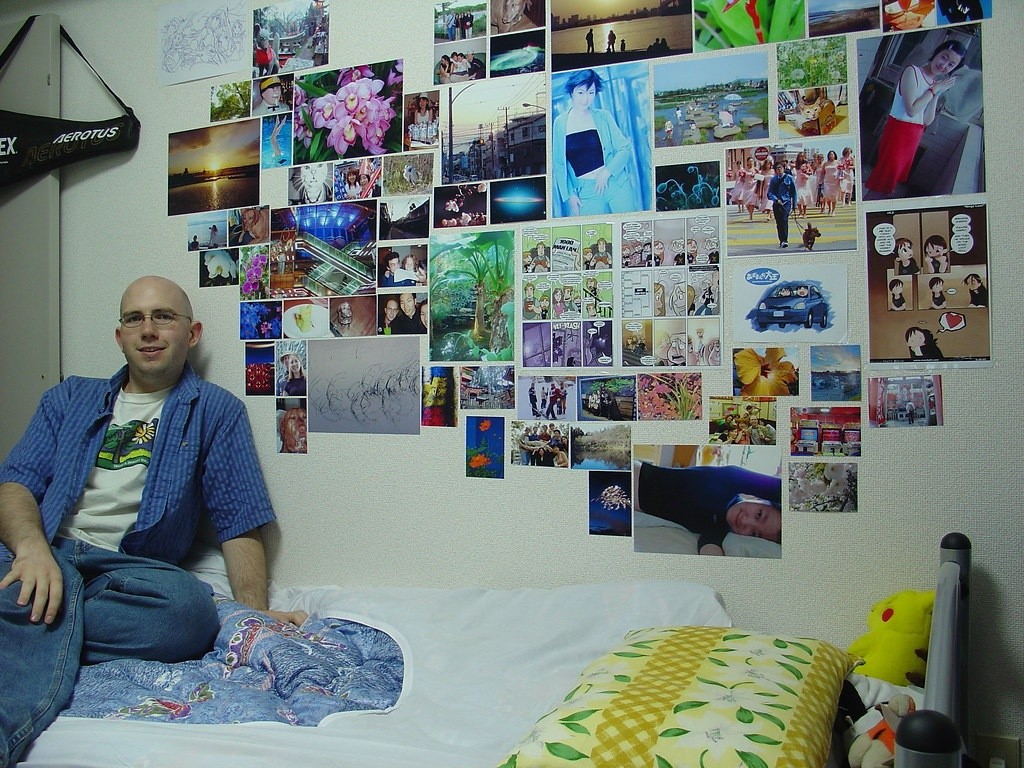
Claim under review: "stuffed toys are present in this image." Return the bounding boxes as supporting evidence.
[843,584,937,688]
[849,692,916,768]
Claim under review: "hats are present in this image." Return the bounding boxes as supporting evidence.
[280,351,291,364]
[258,76,282,93]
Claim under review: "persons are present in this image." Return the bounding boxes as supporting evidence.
[864,40,967,201]
[189,235,199,251]
[410,92,434,126]
[0,275,319,768]
[270,114,287,156]
[343,158,381,200]
[647,37,668,53]
[630,461,782,557]
[254,23,280,76]
[209,224,219,246]
[381,251,426,288]
[276,347,306,397]
[251,77,291,116]
[552,68,638,218]
[438,10,486,84]
[527,382,569,420]
[381,293,428,334]
[729,144,856,250]
[715,401,776,444]
[664,89,742,141]
[905,399,916,423]
[518,423,570,470]
[585,28,616,53]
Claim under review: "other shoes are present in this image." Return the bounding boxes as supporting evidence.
[799,210,806,219]
[847,202,850,207]
[767,214,771,220]
[748,216,752,223]
[842,203,845,207]
[820,208,826,214]
[779,241,789,248]
[737,207,744,212]
[756,206,759,209]
[828,210,835,217]
[816,202,820,207]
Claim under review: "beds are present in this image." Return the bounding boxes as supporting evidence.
[0,533,971,768]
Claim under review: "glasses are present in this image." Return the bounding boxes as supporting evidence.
[118,310,192,328]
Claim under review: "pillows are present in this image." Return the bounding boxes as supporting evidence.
[496,625,866,768]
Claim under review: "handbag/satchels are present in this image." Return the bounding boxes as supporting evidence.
[255,48,275,65]
